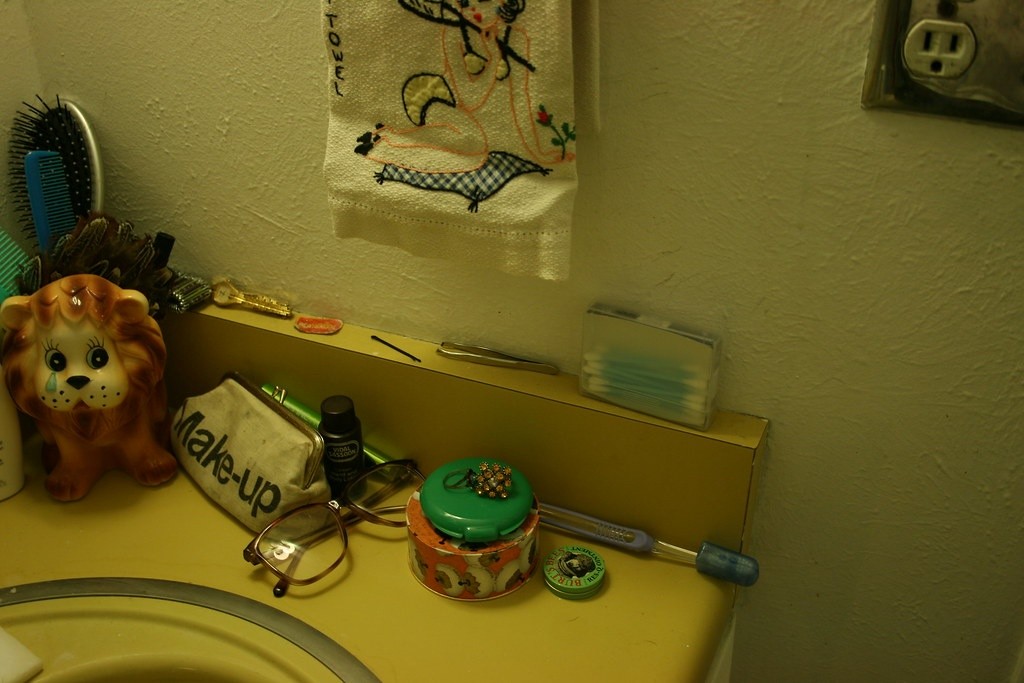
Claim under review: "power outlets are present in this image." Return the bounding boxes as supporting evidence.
[859,0,1024,131]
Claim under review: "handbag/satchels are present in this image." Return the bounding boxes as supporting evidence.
[168,370,332,540]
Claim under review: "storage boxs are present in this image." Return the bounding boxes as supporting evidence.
[577,302,723,432]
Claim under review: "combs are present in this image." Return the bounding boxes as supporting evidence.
[0,93,104,309]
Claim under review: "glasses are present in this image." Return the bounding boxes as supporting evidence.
[242,458,428,598]
